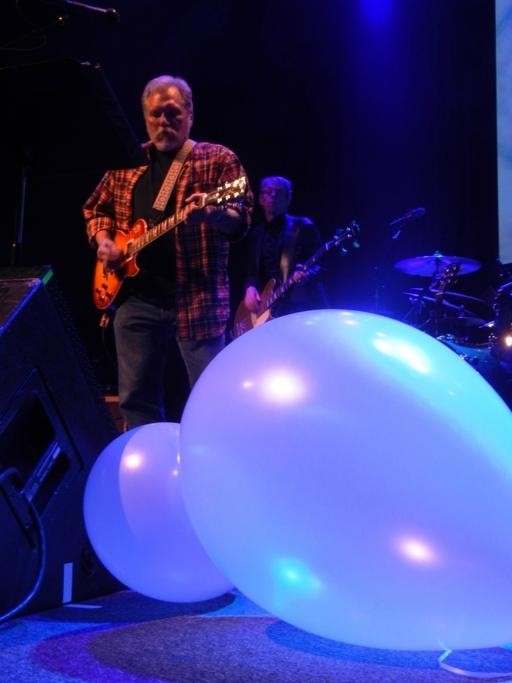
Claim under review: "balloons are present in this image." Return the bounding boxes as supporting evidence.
[171,300,511,655]
[79,418,237,606]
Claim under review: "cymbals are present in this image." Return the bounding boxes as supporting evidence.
[436,291,494,323]
[394,254,480,278]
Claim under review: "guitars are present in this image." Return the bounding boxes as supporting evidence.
[230,219,362,339]
[92,177,247,310]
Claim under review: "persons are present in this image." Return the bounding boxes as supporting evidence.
[225,172,326,321]
[80,72,256,431]
